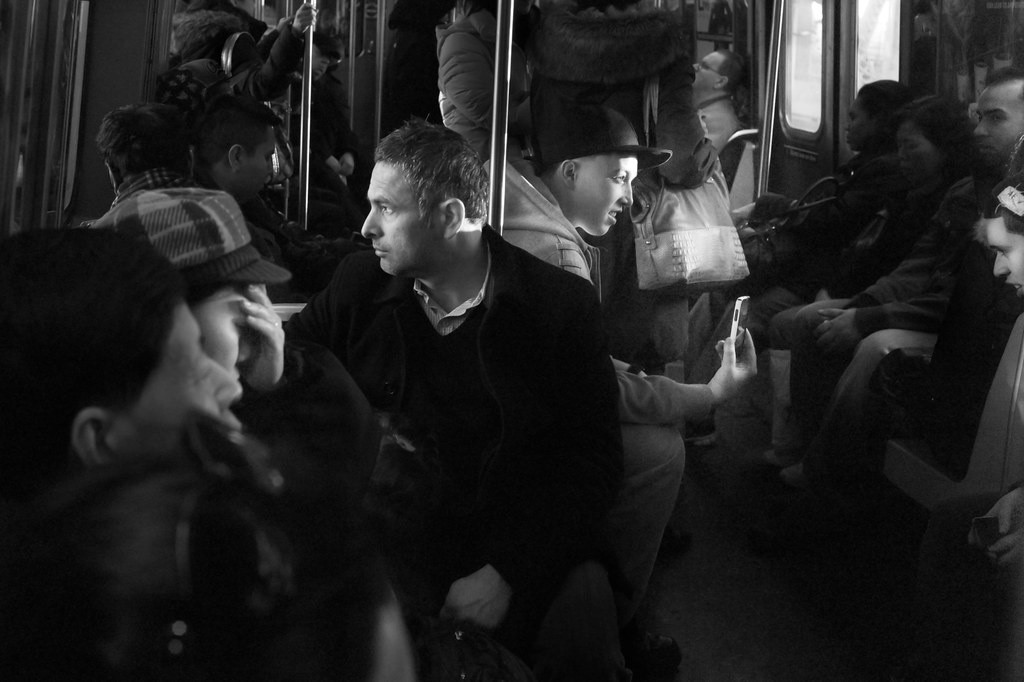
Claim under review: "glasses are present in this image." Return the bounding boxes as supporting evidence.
[699,60,724,77]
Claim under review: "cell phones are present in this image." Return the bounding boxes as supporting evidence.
[972,516,1002,551]
[731,296,750,359]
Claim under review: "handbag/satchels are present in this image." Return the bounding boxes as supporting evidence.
[222,31,293,185]
[627,75,751,288]
[419,616,531,682]
[731,176,846,282]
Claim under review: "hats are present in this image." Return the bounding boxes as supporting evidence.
[90,185,292,284]
[529,107,672,173]
[185,56,232,95]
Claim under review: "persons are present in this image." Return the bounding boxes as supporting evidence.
[1,0,1024,679]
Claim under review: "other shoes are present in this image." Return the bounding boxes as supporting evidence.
[684,416,719,446]
[662,527,691,551]
[778,466,815,488]
[635,635,681,682]
[765,450,796,468]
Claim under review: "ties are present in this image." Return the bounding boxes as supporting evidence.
[316,27,344,62]
